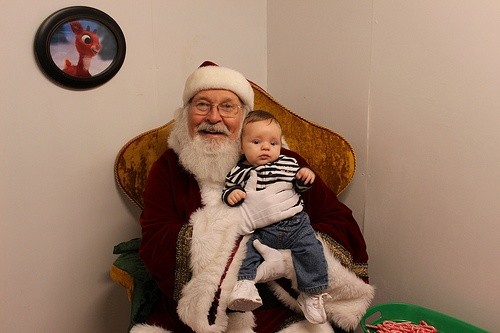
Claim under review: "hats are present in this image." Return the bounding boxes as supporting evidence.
[183,61,254,111]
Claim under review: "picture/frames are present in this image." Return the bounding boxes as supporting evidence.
[36,6,127,92]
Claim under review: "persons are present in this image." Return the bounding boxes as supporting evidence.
[219,111,332,325]
[127,60,375,333]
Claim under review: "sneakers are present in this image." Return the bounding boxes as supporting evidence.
[297,291,328,324]
[228,280,263,312]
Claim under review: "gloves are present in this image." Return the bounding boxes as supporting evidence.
[253,239,288,285]
[234,170,304,240]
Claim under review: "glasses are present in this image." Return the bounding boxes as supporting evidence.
[189,101,243,118]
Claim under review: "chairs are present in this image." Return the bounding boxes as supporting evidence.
[111,82,357,306]
